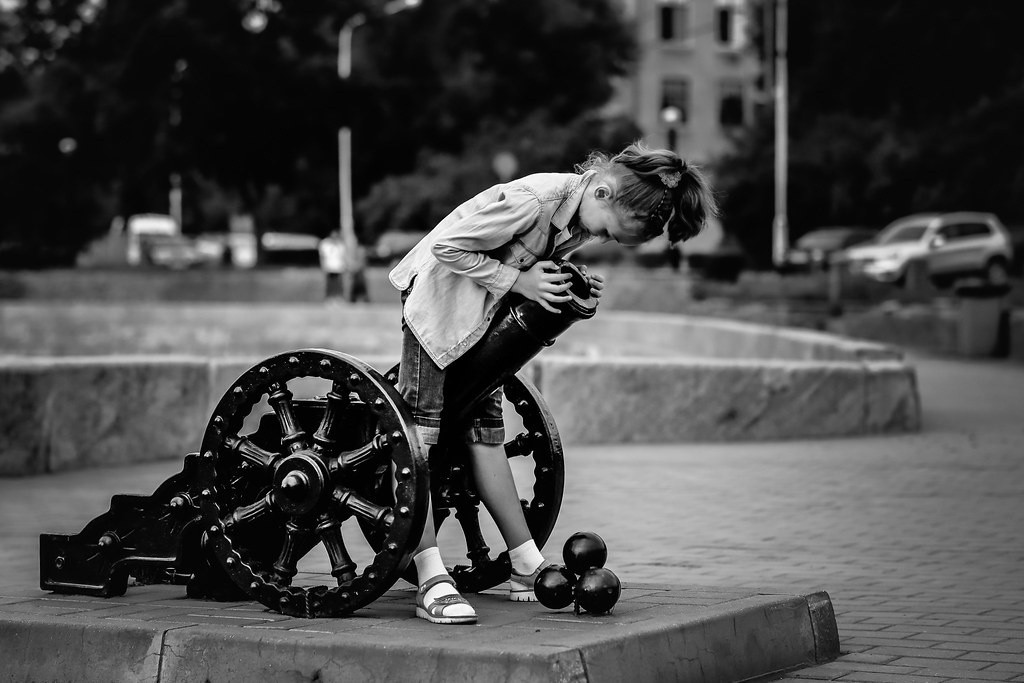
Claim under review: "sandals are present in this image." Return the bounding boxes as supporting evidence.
[508,558,581,602]
[413,573,479,625]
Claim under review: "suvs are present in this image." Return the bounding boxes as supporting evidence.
[827,211,1018,299]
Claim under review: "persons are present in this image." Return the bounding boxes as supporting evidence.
[388,143,719,624]
